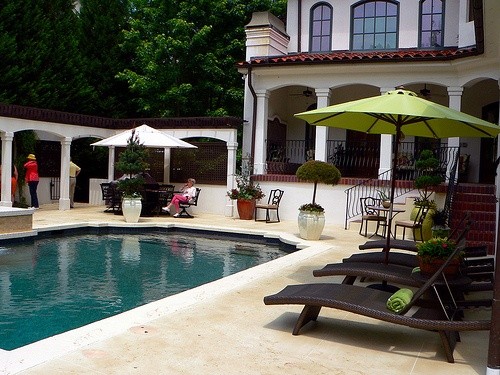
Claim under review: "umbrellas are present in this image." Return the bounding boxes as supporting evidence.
[90,124,198,179]
[294,86,500,292]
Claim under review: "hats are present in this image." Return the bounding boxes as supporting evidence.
[26,154,36,160]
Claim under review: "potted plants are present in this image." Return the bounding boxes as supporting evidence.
[115,121,152,223]
[225,153,266,220]
[415,236,466,279]
[295,160,342,240]
[409,149,442,242]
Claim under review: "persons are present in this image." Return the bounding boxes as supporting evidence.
[162,178,196,216]
[11,156,18,207]
[69,156,81,208]
[24,154,39,209]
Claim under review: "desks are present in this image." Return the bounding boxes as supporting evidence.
[368,206,405,240]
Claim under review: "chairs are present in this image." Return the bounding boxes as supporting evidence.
[254,189,284,224]
[359,197,387,239]
[173,187,201,218]
[263,214,473,365]
[393,204,431,243]
[100,182,175,215]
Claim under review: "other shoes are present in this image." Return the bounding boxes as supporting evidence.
[173,213,180,217]
[28,206,40,210]
[70,205,75,208]
[162,206,170,212]
